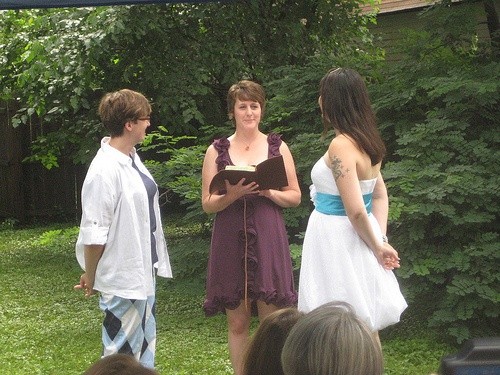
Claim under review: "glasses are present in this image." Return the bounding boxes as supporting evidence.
[136,116,150,123]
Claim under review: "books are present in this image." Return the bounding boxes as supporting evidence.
[208,155,289,200]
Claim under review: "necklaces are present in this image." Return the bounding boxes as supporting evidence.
[236,133,260,151]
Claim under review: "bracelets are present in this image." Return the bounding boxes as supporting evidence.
[382,235,388,242]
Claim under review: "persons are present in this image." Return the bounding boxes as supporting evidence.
[81,300,384,375]
[201,81,302,375]
[75,88,174,375]
[299,67,407,363]
